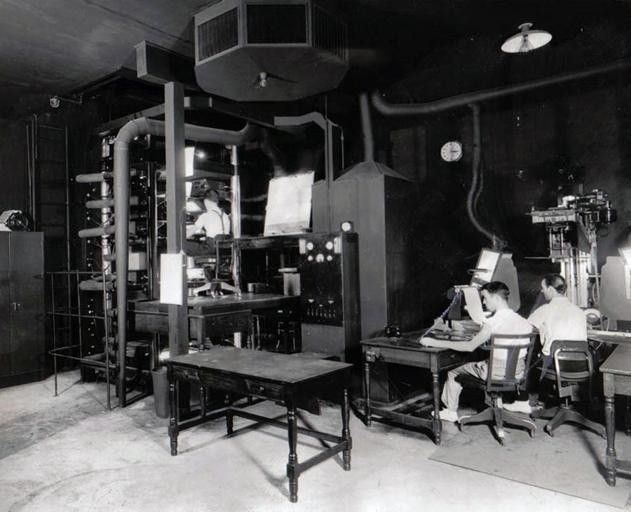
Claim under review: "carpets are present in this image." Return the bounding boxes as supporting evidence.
[429,399,630,509]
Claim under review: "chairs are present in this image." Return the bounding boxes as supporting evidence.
[193,234,238,299]
[530,340,607,440]
[459,328,538,444]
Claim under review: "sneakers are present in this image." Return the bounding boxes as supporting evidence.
[431,408,458,422]
[503,400,532,413]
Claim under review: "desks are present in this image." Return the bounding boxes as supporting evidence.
[237,233,299,288]
[359,325,490,445]
[164,345,354,503]
[600,343,631,486]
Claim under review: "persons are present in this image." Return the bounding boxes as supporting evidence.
[502,272,588,415]
[184,189,230,269]
[418,280,533,423]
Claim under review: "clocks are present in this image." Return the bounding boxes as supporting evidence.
[440,140,464,163]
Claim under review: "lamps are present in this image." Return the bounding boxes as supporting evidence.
[499,20,554,54]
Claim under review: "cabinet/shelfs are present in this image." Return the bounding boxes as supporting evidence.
[1,230,47,389]
[152,170,234,300]
